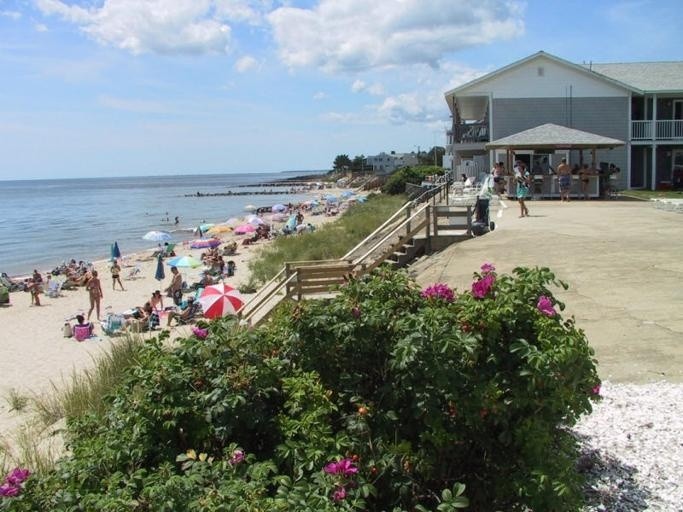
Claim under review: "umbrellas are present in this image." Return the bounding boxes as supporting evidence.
[325,190,367,203]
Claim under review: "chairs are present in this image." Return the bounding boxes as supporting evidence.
[174,304,196,325]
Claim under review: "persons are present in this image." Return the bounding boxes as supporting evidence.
[459,158,621,218]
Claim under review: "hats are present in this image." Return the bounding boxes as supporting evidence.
[152,290,162,298]
